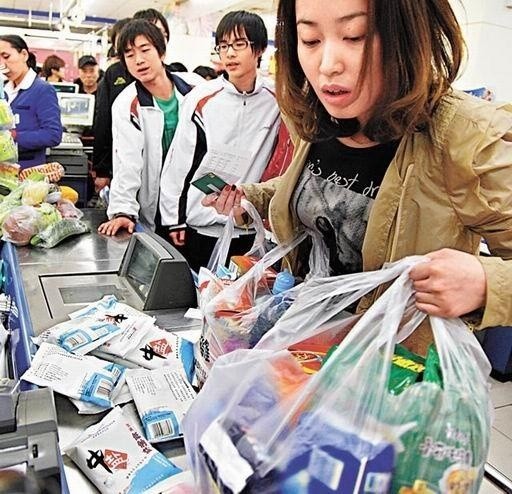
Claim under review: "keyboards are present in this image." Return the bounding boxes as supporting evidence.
[51,135,83,149]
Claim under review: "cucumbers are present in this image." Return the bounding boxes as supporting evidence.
[31,219,83,245]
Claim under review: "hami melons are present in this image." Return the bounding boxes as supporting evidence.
[60,185,78,203]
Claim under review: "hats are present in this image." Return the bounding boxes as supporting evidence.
[79,56,97,67]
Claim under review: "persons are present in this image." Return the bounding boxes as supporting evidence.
[41,55,66,83]
[202,0,512,362]
[90,8,223,236]
[159,11,282,278]
[1,35,62,170]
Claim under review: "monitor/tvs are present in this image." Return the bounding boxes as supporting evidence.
[57,92,95,126]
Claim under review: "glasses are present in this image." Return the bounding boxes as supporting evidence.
[214,40,254,53]
